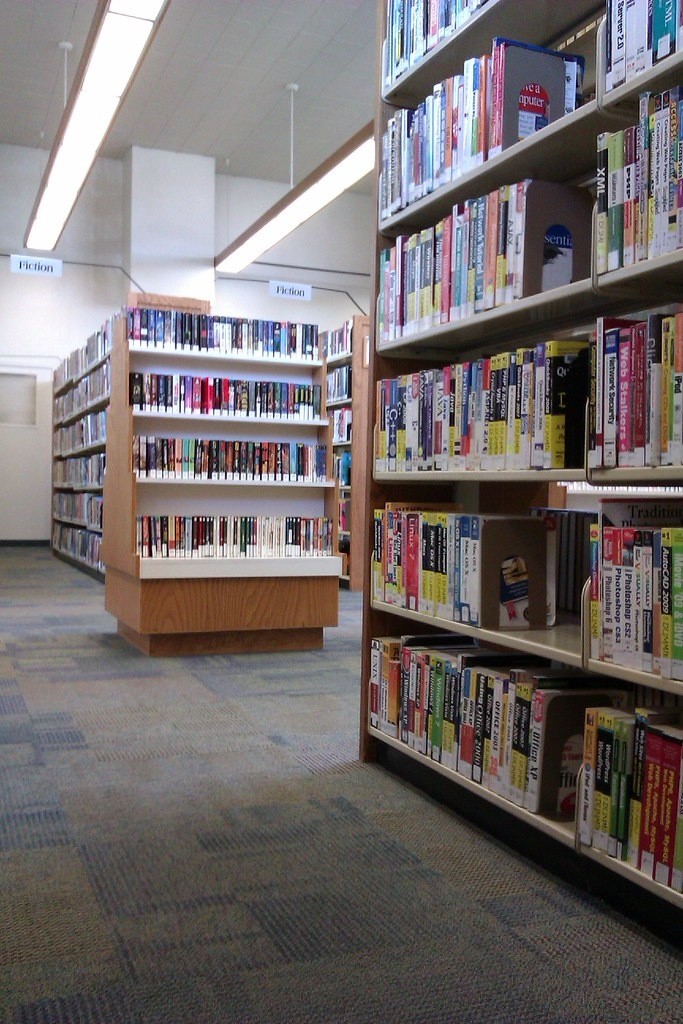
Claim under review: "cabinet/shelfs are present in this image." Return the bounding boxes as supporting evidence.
[354,0,683,952]
[50,307,368,657]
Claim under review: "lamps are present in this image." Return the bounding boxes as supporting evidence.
[23,0,173,251]
[213,119,375,275]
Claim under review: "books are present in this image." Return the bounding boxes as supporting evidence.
[367,0,683,898]
[51,304,353,577]
